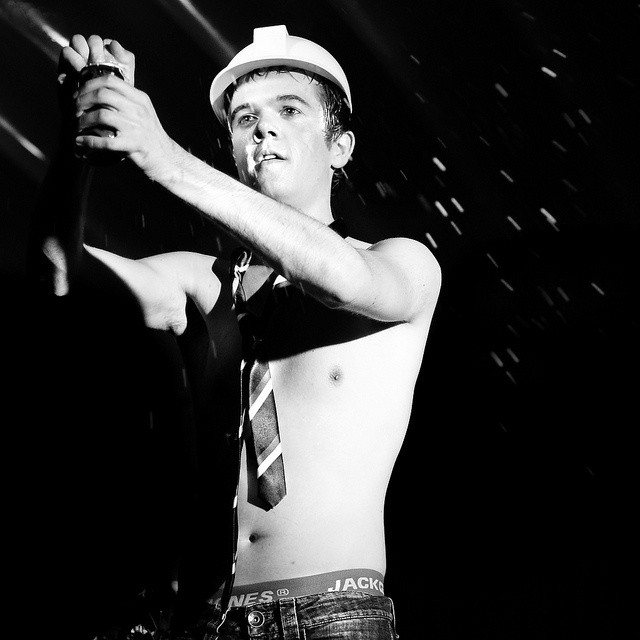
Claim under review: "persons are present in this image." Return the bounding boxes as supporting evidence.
[26,25,442,640]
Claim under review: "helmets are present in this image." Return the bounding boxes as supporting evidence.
[209,25,353,127]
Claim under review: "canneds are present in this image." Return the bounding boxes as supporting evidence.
[73,64,128,163]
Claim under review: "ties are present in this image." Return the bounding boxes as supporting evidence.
[219,251,286,613]
